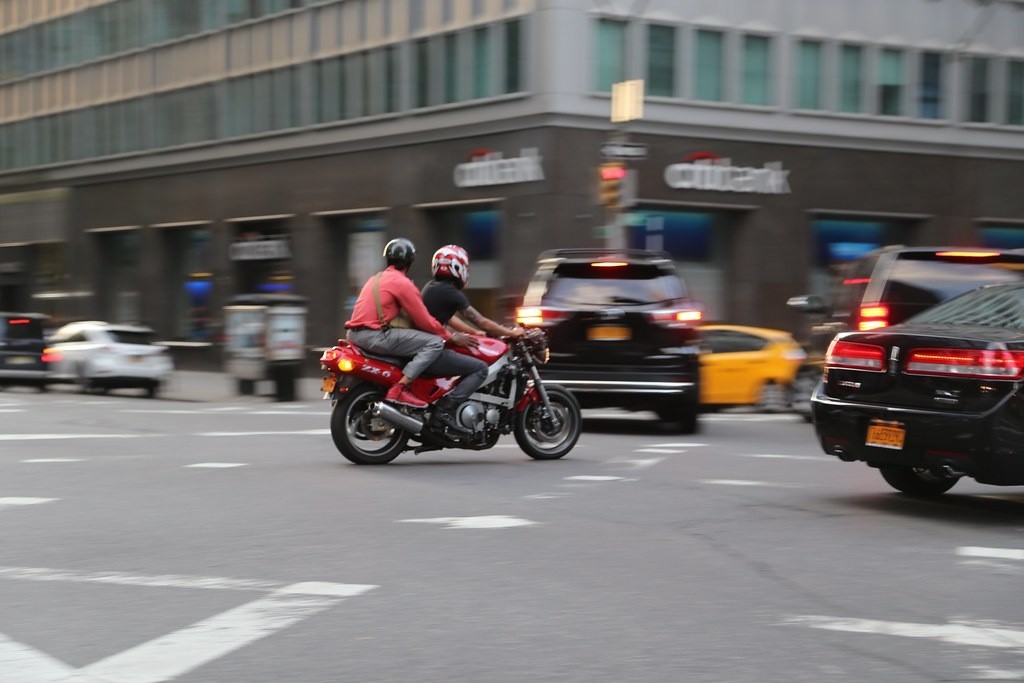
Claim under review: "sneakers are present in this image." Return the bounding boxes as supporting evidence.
[385,383,429,409]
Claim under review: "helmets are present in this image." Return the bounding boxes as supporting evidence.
[431,245,471,290]
[383,237,417,262]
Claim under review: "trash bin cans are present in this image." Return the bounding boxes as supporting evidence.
[265,295,307,380]
[223,293,268,380]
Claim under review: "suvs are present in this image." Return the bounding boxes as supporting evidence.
[853,241,1024,332]
[504,246,704,435]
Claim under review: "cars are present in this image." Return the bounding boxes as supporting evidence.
[692,322,828,425]
[0,283,54,391]
[47,318,177,400]
[809,278,1024,496]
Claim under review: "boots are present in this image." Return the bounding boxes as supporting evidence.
[429,397,474,435]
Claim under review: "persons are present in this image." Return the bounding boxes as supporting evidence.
[345,237,479,408]
[419,244,526,433]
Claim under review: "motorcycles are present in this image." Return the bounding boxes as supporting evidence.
[320,312,583,466]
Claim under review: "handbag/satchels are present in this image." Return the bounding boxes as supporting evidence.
[389,307,414,329]
